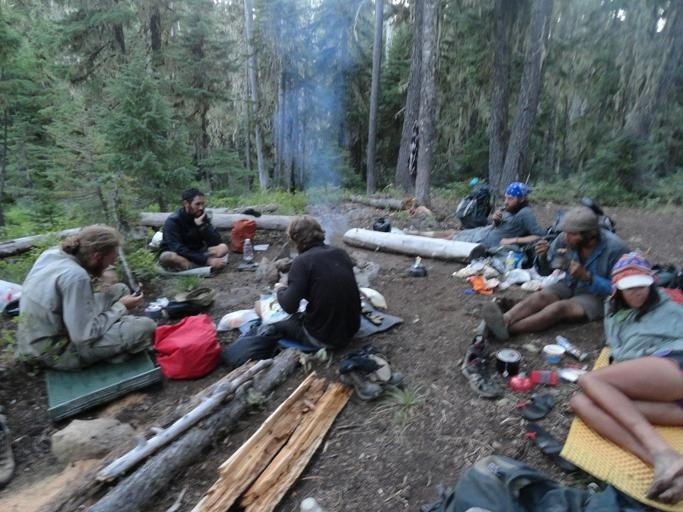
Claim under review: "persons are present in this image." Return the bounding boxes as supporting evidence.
[567,250,683,506]
[14,224,155,373]
[252,214,363,355]
[477,205,631,341]
[155,188,229,276]
[403,181,544,256]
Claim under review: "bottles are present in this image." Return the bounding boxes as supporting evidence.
[100,262,119,293]
[299,495,326,512]
[501,250,516,279]
[408,266,427,276]
[243,238,254,261]
[509,371,531,391]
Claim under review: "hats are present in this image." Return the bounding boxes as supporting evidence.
[606,252,656,306]
[556,206,600,233]
[175,287,216,307]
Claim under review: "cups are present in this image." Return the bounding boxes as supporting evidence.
[542,344,566,365]
[495,349,522,374]
[550,254,571,272]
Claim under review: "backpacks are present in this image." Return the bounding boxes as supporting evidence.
[456,186,494,230]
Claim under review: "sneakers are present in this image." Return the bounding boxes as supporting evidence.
[477,297,514,339]
[339,362,385,401]
[0,423,17,486]
[484,301,510,341]
[460,341,505,397]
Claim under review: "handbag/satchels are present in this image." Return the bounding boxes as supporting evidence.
[651,262,683,290]
[436,454,649,511]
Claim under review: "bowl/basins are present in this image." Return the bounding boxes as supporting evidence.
[144,307,161,318]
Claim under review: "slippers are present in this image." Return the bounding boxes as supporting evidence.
[535,435,576,471]
[522,393,557,422]
[239,260,261,272]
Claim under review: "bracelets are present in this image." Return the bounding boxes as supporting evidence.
[515,237,520,246]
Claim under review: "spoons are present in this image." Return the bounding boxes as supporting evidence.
[543,242,569,256]
